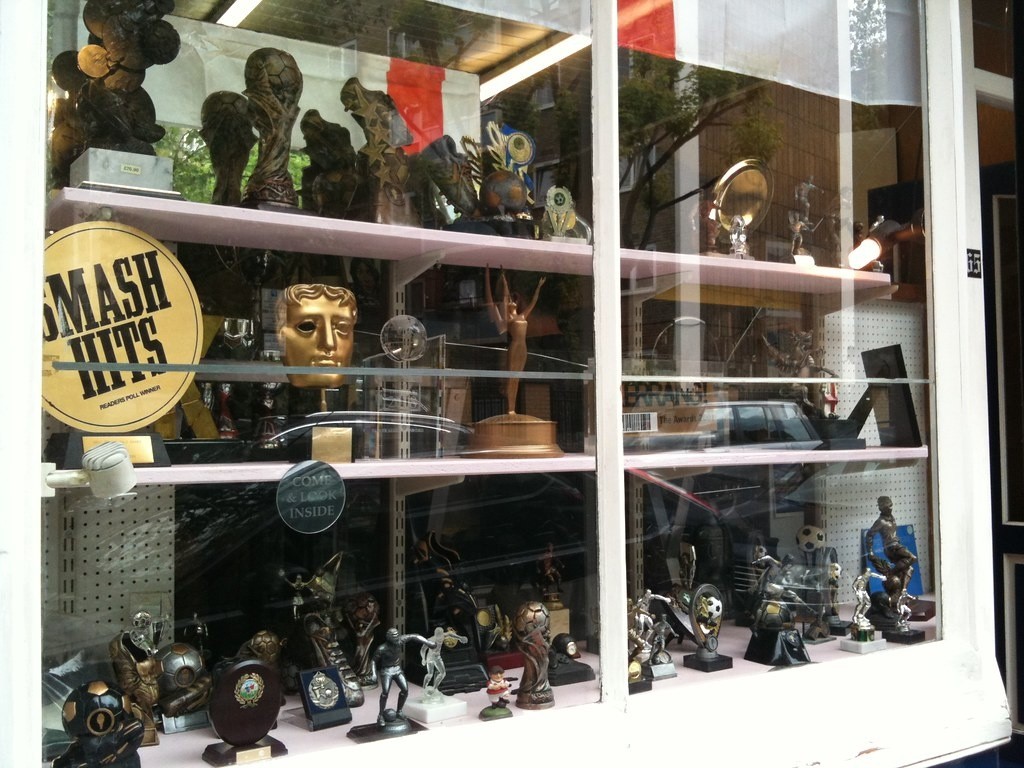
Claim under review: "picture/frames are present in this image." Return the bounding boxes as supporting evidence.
[842,343,924,449]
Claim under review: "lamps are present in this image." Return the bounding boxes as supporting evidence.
[42,441,136,502]
[208,0,264,28]
[847,219,903,273]
[477,30,593,104]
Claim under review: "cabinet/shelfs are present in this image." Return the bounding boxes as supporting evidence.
[42,184,929,486]
